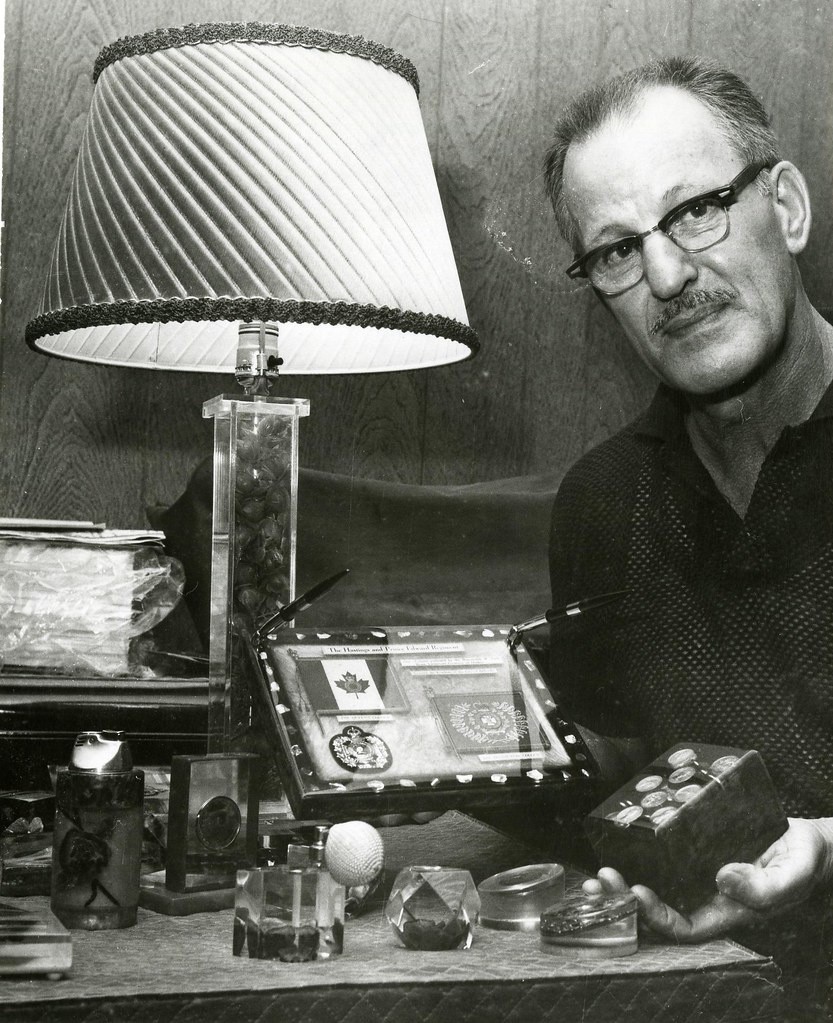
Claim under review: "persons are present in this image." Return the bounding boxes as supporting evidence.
[358,57,833,1023]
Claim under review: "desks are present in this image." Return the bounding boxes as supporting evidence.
[0,809,787,1023]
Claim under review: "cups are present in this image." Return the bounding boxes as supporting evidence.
[50,768,145,932]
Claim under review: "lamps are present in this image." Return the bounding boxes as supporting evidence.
[25,23,479,842]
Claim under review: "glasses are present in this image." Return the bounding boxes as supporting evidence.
[565,157,778,297]
